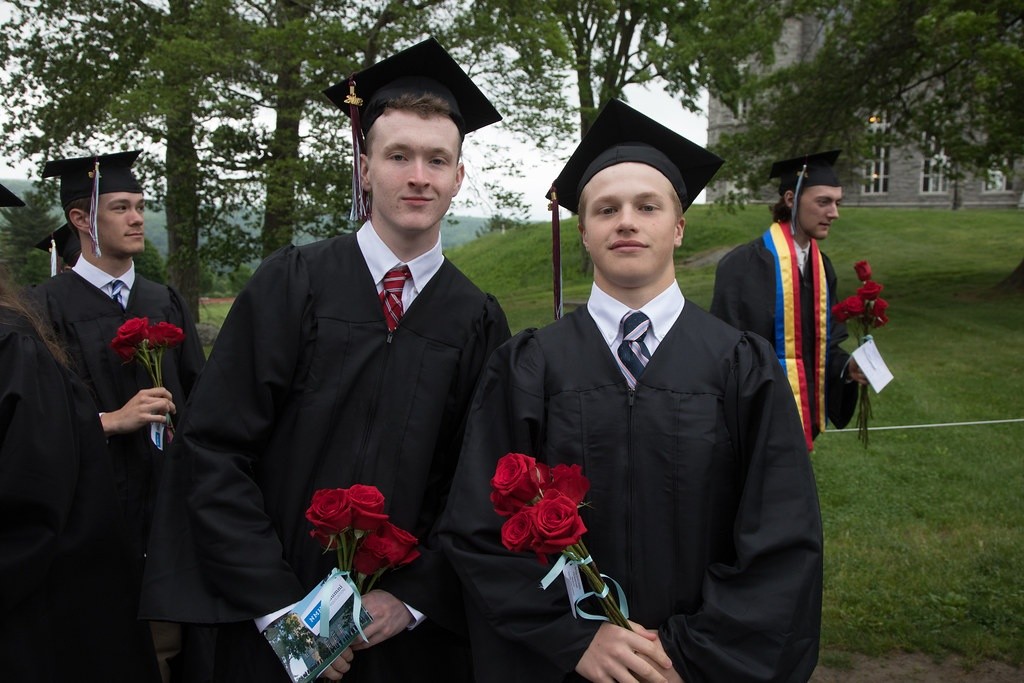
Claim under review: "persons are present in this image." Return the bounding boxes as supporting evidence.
[437,145,823,683]
[170,86,512,683]
[711,158,868,451]
[0,165,207,683]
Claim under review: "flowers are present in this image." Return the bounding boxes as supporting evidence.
[833,260,889,448]
[305,482,422,600]
[111,315,186,435]
[491,451,634,633]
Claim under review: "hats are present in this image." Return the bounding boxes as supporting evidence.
[769,149,843,236]
[545,97,726,321]
[0,184,26,207]
[33,222,82,277]
[322,37,503,221]
[42,149,144,257]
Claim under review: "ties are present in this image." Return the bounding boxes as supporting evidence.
[109,279,124,306]
[614,310,652,393]
[378,266,413,331]
